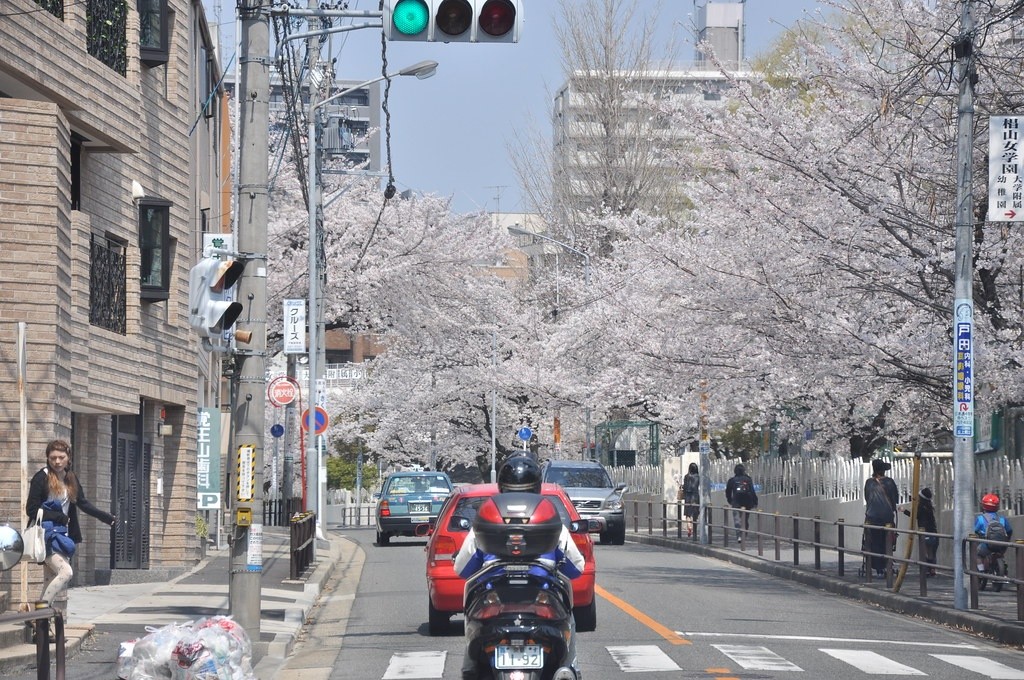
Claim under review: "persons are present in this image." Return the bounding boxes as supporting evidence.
[726,464,758,543]
[864,459,899,578]
[452,456,584,680]
[975,494,1012,584]
[898,488,939,577]
[682,463,699,537]
[26,441,116,644]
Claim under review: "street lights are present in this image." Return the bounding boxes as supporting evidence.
[306,60,440,510]
[508,226,592,461]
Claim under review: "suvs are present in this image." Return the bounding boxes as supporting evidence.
[539,458,630,545]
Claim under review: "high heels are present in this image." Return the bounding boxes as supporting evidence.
[688,529,692,537]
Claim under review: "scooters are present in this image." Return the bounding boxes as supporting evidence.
[448,494,592,680]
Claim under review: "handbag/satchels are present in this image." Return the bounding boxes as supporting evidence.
[677,485,686,500]
[20,508,47,563]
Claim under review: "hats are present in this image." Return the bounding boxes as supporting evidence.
[919,487,932,502]
[872,459,891,470]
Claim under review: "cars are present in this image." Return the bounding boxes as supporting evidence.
[415,481,604,634]
[372,471,456,546]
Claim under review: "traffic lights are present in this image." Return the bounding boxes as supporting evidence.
[189,256,243,337]
[383,0,525,43]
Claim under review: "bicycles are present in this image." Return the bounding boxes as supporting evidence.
[975,530,1005,592]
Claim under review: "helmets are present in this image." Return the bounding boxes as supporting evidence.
[497,451,544,494]
[982,494,1000,511]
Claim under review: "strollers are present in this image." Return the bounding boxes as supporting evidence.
[858,509,899,577]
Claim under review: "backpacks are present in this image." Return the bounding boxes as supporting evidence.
[982,513,1008,553]
[731,477,752,506]
